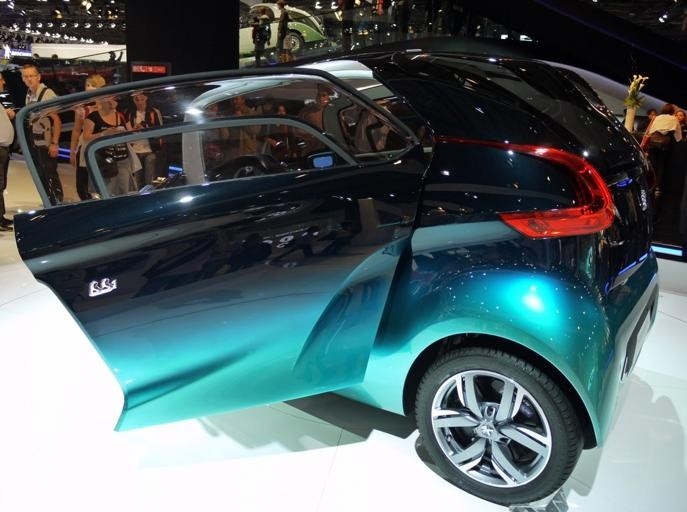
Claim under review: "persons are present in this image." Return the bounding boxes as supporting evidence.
[249,0,483,67]
[1,63,337,232]
[620,102,686,226]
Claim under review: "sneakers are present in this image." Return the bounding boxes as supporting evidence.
[654,190,661,196]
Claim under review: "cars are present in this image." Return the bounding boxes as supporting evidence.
[11,46,661,507]
[236,2,325,57]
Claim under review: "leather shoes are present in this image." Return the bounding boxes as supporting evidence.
[0,219,13,231]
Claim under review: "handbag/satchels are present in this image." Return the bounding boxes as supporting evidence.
[100,157,119,177]
